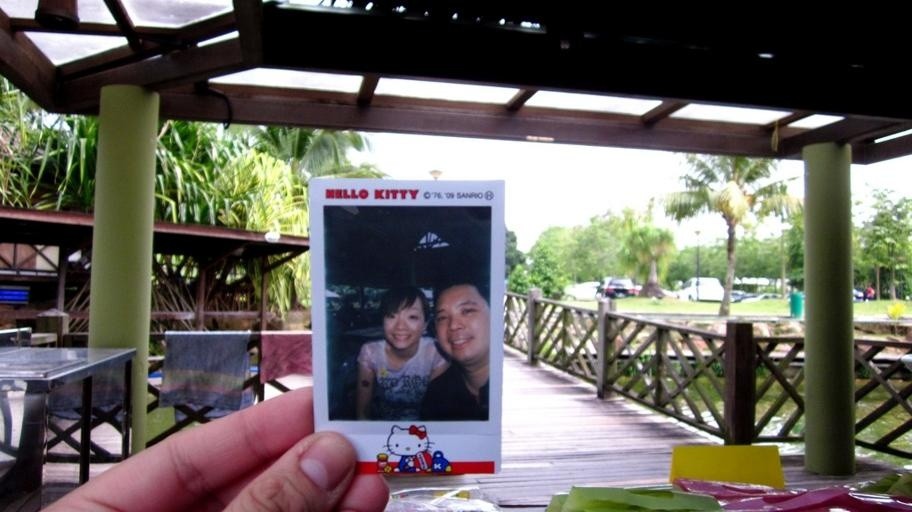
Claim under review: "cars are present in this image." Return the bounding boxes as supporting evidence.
[677,278,780,302]
[564,277,639,301]
[853,287,868,302]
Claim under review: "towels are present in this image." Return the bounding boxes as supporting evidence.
[157,327,313,425]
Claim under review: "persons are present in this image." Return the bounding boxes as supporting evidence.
[38,387,390,512]
[354,286,448,420]
[420,273,491,421]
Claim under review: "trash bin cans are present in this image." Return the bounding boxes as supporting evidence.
[791,293,803,318]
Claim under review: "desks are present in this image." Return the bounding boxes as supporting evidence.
[1,344,137,484]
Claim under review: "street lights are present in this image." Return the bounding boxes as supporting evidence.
[695,230,700,301]
